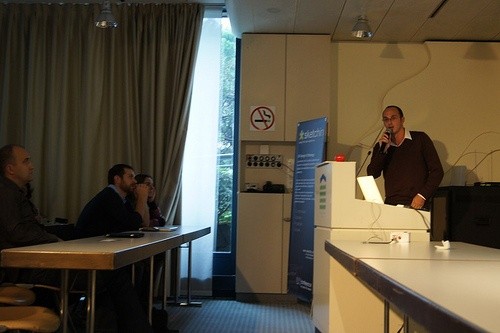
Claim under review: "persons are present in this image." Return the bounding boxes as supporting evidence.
[0,144,79,322]
[367,105,445,212]
[76,164,165,325]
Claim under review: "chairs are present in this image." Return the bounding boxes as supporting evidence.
[0,307,61,333]
[0,287,36,306]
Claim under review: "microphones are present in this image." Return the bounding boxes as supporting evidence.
[380,129,392,151]
[357,151,371,176]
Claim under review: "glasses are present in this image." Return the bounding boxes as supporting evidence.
[382,116,401,122]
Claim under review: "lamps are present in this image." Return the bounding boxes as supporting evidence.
[352,15,374,37]
[95,1,118,29]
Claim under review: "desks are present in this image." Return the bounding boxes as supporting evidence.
[0,226,212,333]
[325,243,500,333]
